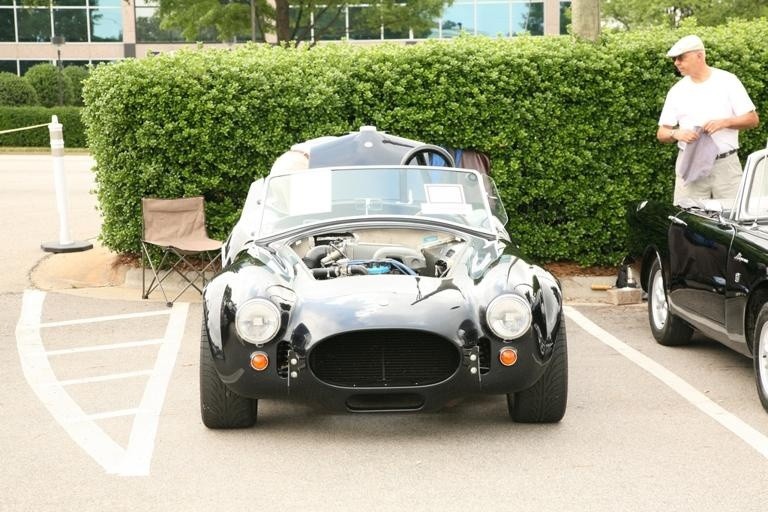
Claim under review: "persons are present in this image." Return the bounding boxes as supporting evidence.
[656,35,762,208]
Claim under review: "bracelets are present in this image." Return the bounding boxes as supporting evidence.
[670,129,677,140]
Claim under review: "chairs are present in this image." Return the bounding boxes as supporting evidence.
[137,193,222,311]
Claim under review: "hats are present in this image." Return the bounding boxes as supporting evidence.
[666,35,704,57]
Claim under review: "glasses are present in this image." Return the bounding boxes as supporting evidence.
[672,55,683,61]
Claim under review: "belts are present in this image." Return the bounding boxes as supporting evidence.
[716,149,736,159]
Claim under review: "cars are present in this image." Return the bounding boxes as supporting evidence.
[634,148,766,413]
[195,125,569,431]
